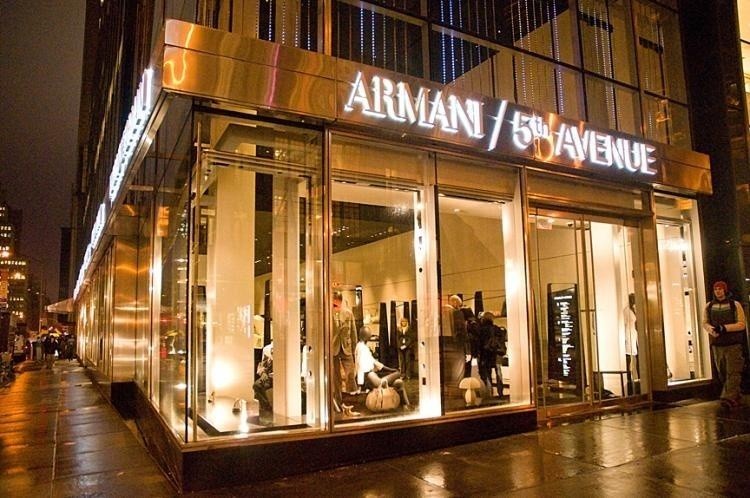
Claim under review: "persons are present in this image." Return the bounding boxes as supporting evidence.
[702,281,747,410]
[440,295,472,387]
[349,326,410,411]
[331,290,354,412]
[253,336,272,409]
[476,312,500,382]
[623,292,640,382]
[39,329,74,370]
[395,317,413,381]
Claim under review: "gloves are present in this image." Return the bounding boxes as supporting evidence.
[713,320,726,334]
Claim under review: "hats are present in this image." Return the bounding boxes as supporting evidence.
[714,281,727,291]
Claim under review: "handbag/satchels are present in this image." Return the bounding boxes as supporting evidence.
[366,379,401,411]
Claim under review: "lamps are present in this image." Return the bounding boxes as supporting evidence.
[457,376,485,407]
